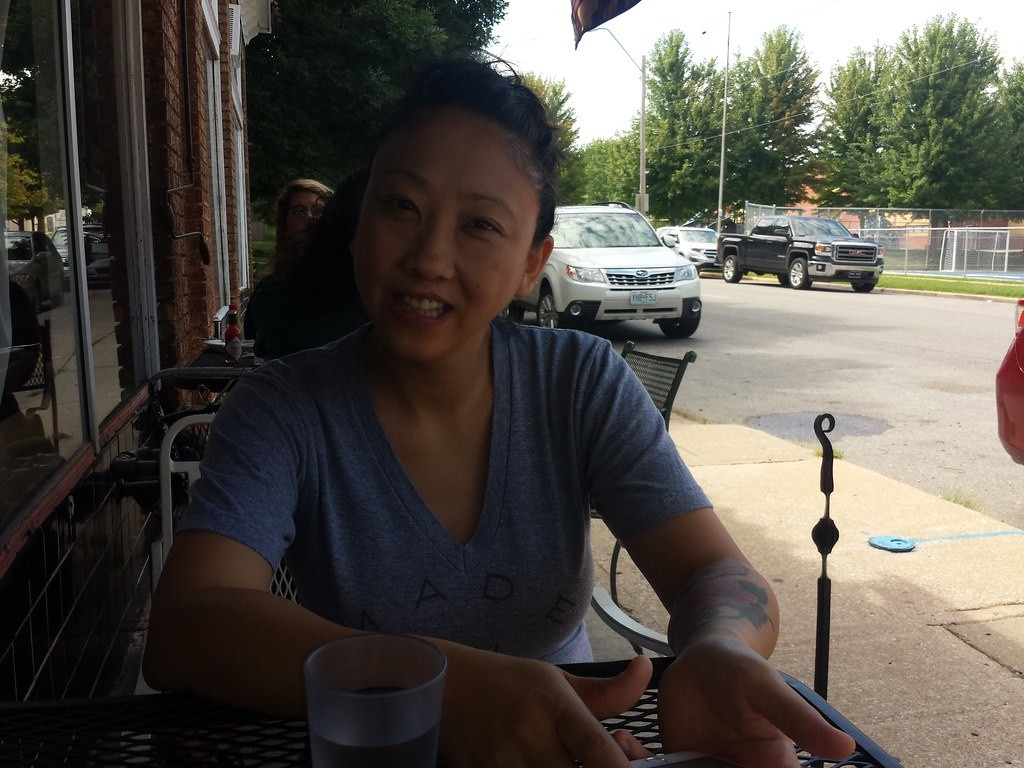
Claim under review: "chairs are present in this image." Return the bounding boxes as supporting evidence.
[587,341,697,524]
[0,317,62,466]
[148,366,256,462]
[134,412,680,699]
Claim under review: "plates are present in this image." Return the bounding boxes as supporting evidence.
[204,340,255,348]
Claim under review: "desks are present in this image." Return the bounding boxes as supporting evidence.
[174,341,268,396]
[0,653,905,768]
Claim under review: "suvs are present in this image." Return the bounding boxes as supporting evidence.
[496,200,702,340]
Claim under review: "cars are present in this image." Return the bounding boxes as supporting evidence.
[654,224,725,277]
[4,231,65,309]
[51,224,112,291]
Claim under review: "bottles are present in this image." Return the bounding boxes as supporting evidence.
[225,304,242,364]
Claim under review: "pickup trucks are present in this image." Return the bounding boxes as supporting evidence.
[716,215,886,295]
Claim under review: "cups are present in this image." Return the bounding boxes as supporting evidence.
[304,634,448,767]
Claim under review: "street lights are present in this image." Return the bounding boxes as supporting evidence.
[586,27,647,219]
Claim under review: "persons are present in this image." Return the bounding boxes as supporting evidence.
[266,155,370,360]
[243,178,335,340]
[0,280,41,419]
[142,62,854,768]
